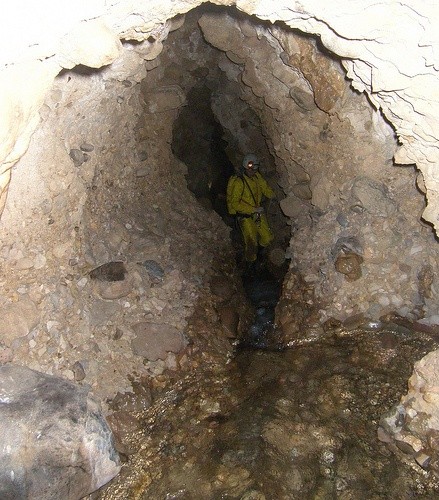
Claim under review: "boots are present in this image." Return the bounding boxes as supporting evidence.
[257,244,266,265]
[244,261,258,278]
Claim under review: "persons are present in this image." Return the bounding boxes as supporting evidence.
[226,155,280,270]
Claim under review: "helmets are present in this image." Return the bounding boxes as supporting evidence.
[242,154,259,171]
